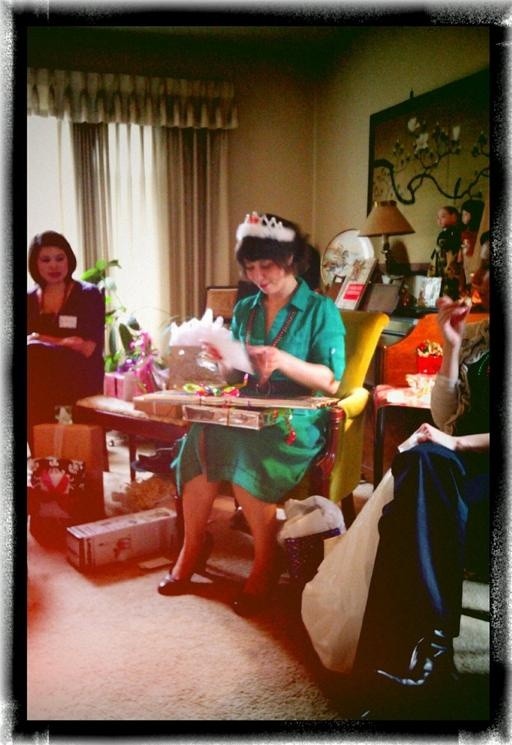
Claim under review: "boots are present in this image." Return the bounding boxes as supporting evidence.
[376,626,463,701]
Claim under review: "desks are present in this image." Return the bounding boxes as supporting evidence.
[359,312,426,484]
[72,394,191,484]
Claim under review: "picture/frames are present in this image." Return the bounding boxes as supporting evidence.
[368,67,492,272]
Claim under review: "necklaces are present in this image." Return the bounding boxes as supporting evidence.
[244,306,299,380]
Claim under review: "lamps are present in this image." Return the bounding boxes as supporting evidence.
[358,200,417,274]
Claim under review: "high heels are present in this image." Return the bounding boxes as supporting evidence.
[158,531,214,596]
[233,542,287,618]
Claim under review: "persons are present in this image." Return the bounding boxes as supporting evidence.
[157,209,346,617]
[27,230,109,553]
[426,198,486,301]
[299,225,491,698]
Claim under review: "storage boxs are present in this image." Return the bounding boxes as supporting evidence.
[64,505,178,569]
[104,371,142,400]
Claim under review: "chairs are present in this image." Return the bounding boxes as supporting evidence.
[309,309,389,529]
